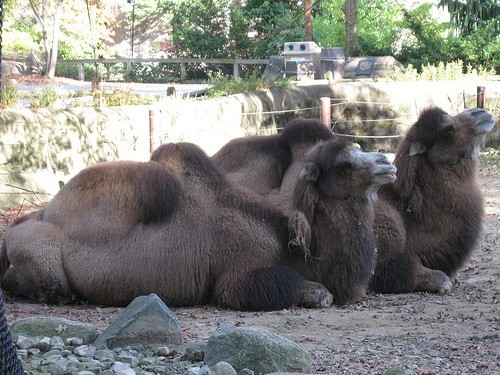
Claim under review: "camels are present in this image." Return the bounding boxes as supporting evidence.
[1,136,397,310]
[210,107,496,294]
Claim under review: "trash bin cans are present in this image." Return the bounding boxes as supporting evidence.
[320,46,345,79]
[282,41,321,81]
